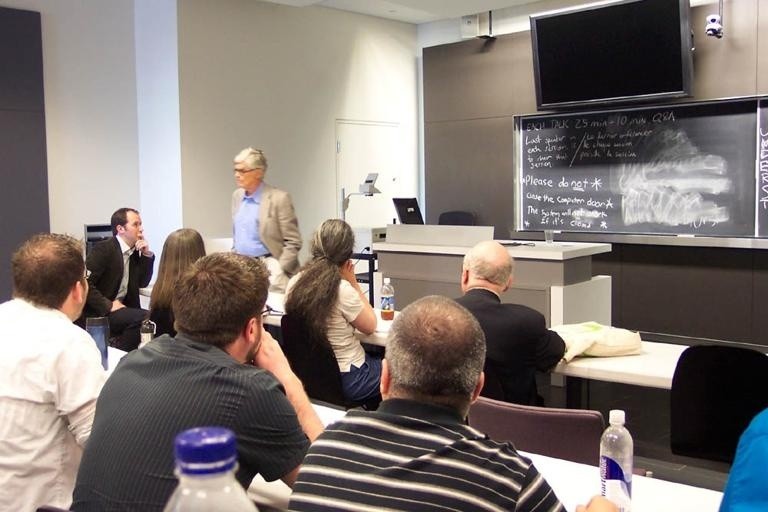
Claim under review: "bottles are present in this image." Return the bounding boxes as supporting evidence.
[86,317,109,370]
[140,318,155,351]
[380,278,394,321]
[162,424,258,512]
[597,410,633,511]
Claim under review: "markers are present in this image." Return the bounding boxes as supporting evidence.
[553,231,561,234]
[677,235,695,237]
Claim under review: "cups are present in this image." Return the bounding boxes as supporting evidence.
[544,229,553,246]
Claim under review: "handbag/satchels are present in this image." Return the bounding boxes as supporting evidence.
[547,320,642,363]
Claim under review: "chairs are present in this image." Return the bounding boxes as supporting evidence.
[280,313,371,413]
[670,346,768,463]
[462,397,605,468]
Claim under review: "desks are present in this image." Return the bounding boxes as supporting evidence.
[140,282,768,417]
[91,345,723,511]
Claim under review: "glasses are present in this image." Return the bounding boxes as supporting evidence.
[83,269,92,280]
[234,168,256,174]
[260,305,272,317]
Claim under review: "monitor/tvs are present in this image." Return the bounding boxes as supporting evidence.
[391,196,425,224]
[528,1,697,112]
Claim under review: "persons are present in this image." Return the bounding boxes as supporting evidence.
[288,294,621,512]
[149,229,206,338]
[70,252,326,512]
[719,408,768,512]
[0,232,109,512]
[231,144,303,294]
[452,240,566,407]
[72,208,156,352]
[282,219,384,406]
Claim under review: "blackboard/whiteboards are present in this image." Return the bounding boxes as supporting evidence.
[511,95,768,249]
